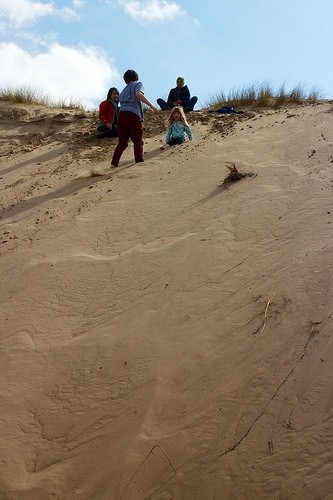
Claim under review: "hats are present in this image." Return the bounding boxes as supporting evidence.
[177,77,184,85]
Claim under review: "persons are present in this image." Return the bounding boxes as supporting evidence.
[157,77,198,113]
[97,87,119,139]
[161,107,193,150]
[110,69,159,168]
